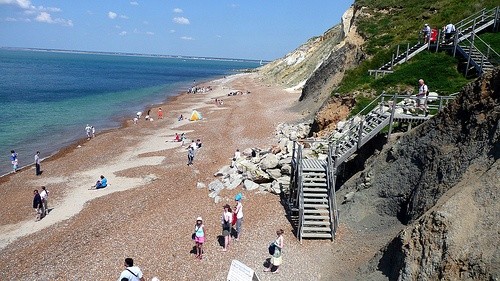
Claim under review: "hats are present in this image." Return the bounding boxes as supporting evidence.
[197,217,202,220]
[222,204,230,208]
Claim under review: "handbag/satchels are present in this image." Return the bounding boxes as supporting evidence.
[273,247,281,258]
[192,233,196,239]
[223,219,230,231]
[269,243,276,255]
[232,213,237,226]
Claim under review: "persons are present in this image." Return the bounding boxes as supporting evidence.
[231,157,236,168]
[33,151,43,175]
[186,138,202,165]
[222,205,232,252]
[178,115,183,122]
[187,86,209,94]
[264,229,284,274]
[414,79,429,116]
[32,186,50,222]
[11,150,19,174]
[174,132,184,142]
[232,193,243,241]
[147,110,151,116]
[88,175,108,190]
[193,217,206,259]
[422,22,456,44]
[132,117,138,125]
[117,258,144,281]
[158,107,163,119]
[235,149,240,159]
[85,124,96,141]
[137,112,142,119]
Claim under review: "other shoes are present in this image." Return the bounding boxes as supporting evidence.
[264,268,271,271]
[273,271,280,274]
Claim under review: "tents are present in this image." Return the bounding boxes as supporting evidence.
[190,110,202,121]
